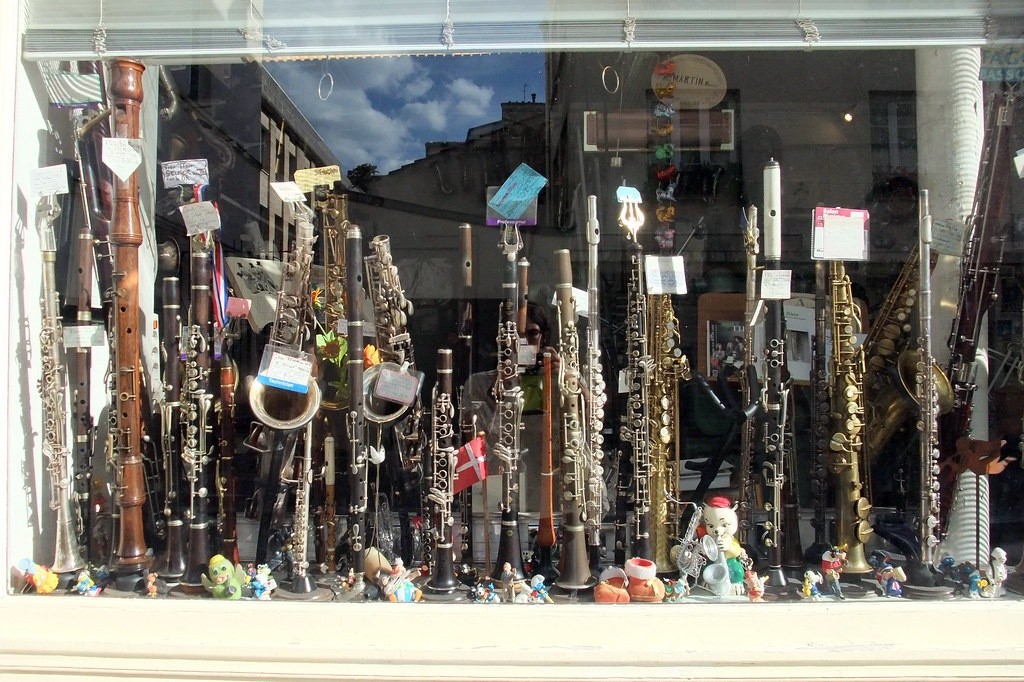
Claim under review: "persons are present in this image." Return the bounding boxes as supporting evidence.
[500,561,516,603]
[461,300,585,559]
[803,545,1009,601]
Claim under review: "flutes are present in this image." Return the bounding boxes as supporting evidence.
[22,52,1014,595]
